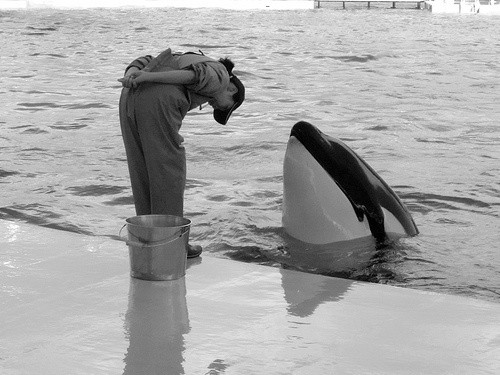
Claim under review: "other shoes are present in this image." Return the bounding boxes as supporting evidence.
[187,244,202,258]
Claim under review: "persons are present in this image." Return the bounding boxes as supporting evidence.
[116,46,245,258]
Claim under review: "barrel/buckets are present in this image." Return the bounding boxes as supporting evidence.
[125,276,192,333]
[118,214,192,281]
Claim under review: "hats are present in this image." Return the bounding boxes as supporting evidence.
[213,76,244,125]
